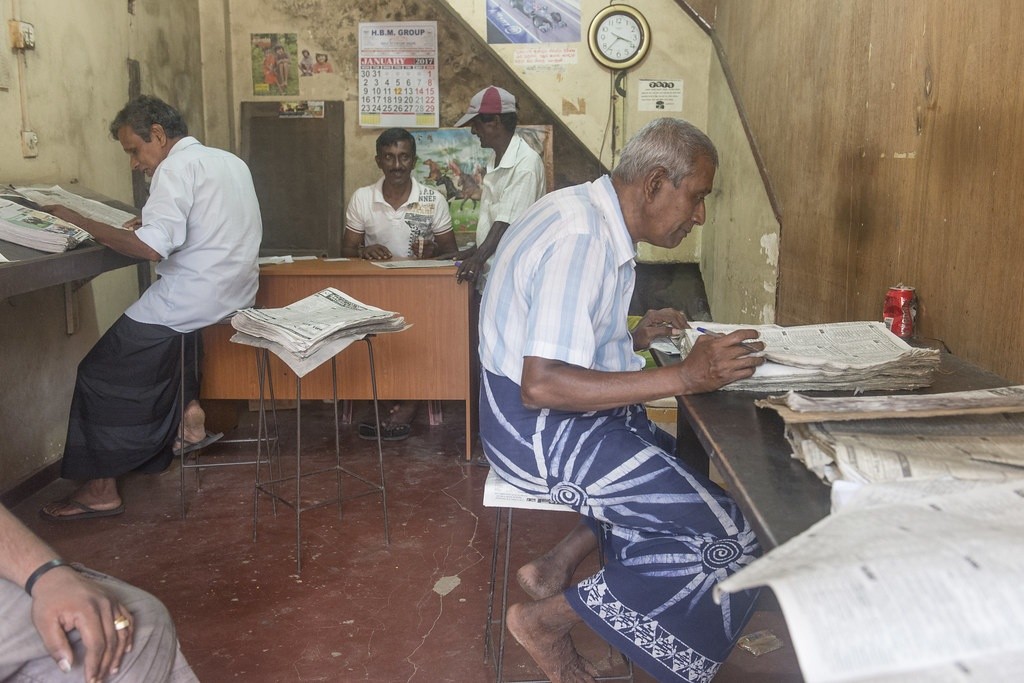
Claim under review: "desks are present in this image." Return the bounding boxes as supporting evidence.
[198,256,477,460]
[647,336,1021,557]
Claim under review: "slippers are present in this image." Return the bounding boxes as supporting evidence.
[39,497,124,520]
[171,428,224,456]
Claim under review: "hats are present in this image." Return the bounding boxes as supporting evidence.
[453,85,515,128]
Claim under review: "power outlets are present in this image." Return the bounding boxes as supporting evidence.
[21,131,39,158]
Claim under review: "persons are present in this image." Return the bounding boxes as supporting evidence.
[313,54,333,73]
[40,95,263,520]
[297,50,314,76]
[431,84,547,312]
[262,47,283,93]
[340,128,459,441]
[275,45,292,87]
[478,117,768,682]
[0,502,200,683]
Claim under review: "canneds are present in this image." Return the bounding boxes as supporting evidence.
[883,282,917,342]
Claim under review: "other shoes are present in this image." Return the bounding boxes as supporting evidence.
[359,416,410,441]
[476,435,490,466]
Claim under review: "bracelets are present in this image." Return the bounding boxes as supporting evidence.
[415,236,424,258]
[354,243,365,259]
[25,559,81,595]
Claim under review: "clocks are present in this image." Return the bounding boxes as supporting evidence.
[587,4,650,69]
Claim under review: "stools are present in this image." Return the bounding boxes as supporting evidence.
[180,306,284,519]
[483,463,634,683]
[253,333,391,574]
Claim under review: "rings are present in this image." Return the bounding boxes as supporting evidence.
[461,272,467,275]
[469,270,473,274]
[113,616,130,631]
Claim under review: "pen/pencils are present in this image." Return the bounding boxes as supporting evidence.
[698,326,758,355]
